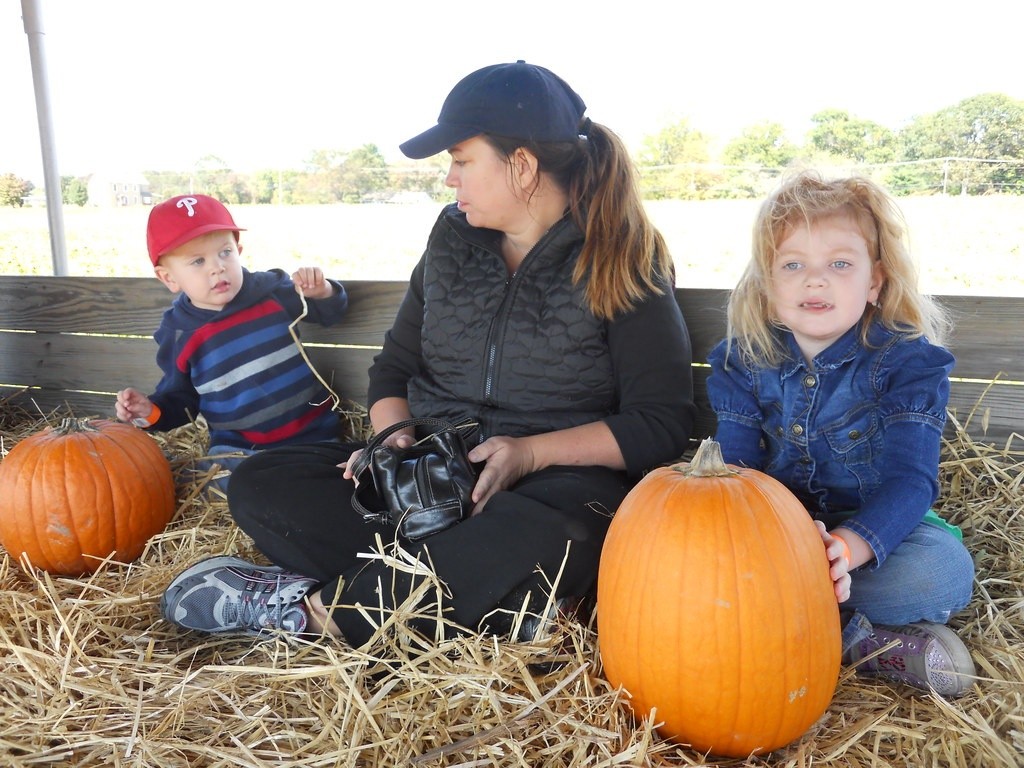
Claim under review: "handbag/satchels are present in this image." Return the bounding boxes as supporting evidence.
[349,415,487,541]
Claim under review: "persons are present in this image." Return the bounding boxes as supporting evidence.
[708,172,980,700]
[113,195,349,495]
[162,61,698,646]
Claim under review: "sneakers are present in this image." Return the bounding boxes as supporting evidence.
[160,556,320,644]
[849,621,977,697]
[518,596,586,669]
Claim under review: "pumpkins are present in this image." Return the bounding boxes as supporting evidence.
[596,435,841,759]
[0,415,174,577]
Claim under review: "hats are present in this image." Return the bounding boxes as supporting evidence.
[399,60,585,159]
[147,195,247,267]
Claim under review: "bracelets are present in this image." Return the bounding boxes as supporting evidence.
[828,531,851,572]
[131,403,161,427]
